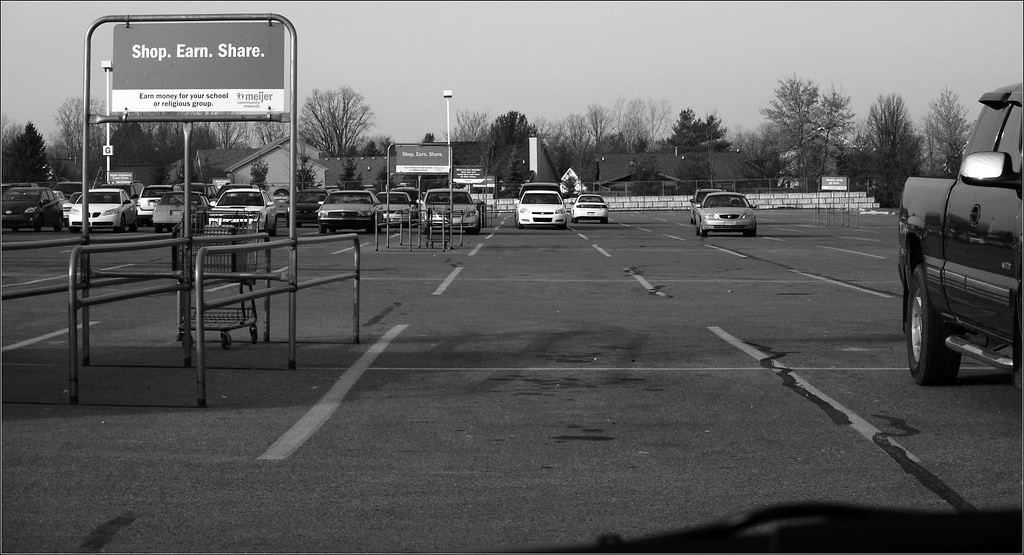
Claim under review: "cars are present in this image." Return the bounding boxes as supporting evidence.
[1,180,205,235]
[286,187,422,234]
[571,194,609,223]
[204,188,282,237]
[422,189,481,234]
[514,183,567,229]
[689,189,757,237]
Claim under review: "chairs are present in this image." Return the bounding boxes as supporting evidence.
[430,196,442,202]
[731,199,740,207]
[170,197,179,203]
[398,197,406,202]
[454,196,466,202]
[318,195,325,201]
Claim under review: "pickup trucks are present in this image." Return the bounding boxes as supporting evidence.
[898,82,1024,386]
